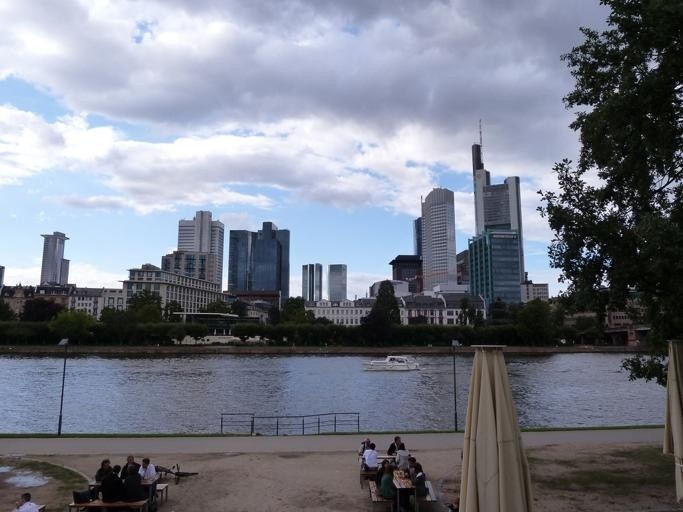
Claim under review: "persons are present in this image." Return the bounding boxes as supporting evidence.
[11,491,39,511]
[358,431,430,512]
[119,455,141,479]
[122,466,149,502]
[139,456,158,497]
[99,464,123,504]
[94,458,113,483]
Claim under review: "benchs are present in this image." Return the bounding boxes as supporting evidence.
[156,483,168,505]
[71,500,148,512]
[360,470,379,489]
[423,480,437,502]
[368,480,394,512]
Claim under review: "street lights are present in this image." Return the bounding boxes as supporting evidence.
[58,339,69,433]
[452,339,461,433]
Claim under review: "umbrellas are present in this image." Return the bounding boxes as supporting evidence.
[662,335,683,504]
[455,340,535,511]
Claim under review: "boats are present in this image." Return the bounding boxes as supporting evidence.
[361,356,420,371]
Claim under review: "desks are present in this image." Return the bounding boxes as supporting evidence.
[393,471,417,512]
[361,455,412,459]
[88,481,155,501]
